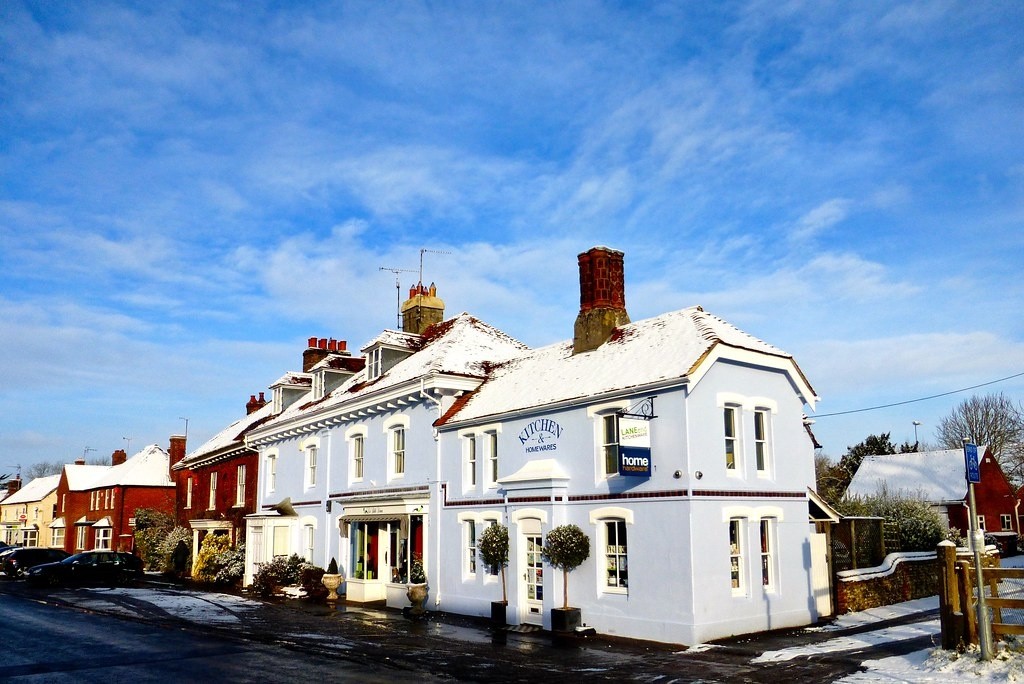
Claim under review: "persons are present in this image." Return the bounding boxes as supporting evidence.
[170,540,190,571]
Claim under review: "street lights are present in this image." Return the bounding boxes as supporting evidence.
[912,421,922,451]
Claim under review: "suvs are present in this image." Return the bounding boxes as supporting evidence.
[0,540,76,583]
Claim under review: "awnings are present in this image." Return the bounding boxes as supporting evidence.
[808,486,844,524]
[339,514,408,539]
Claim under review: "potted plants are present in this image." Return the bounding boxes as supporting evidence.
[405,561,428,615]
[476,521,513,626]
[543,524,591,633]
[321,557,345,600]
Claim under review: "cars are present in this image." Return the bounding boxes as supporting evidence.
[26,549,136,590]
